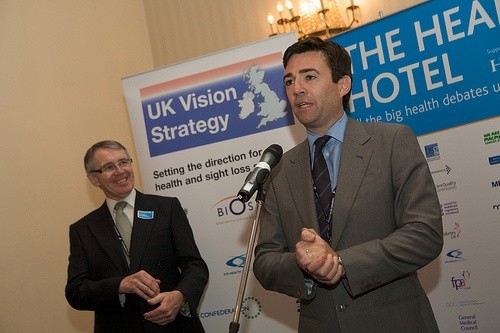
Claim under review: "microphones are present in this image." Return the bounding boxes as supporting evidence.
[237,144,284,203]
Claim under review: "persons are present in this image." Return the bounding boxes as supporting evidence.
[65,141,210,333]
[253,36,445,333]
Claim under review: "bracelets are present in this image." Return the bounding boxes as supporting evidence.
[336,254,347,271]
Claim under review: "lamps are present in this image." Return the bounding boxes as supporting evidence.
[267,0,361,43]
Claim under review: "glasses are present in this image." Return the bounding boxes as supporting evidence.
[89,157,133,174]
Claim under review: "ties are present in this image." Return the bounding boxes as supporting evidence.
[114,201,133,272]
[312,134,332,245]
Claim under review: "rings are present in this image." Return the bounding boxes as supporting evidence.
[304,249,311,256]
